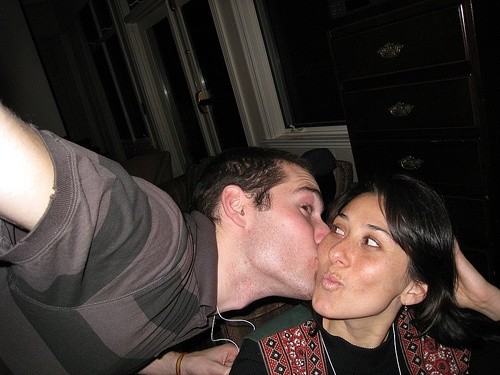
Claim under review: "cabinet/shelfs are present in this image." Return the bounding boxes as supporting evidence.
[323,0,500,287]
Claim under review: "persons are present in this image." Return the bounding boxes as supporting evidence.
[224,170,500,375]
[0,100,332,375]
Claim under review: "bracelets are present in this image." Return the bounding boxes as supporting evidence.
[170,353,188,374]
